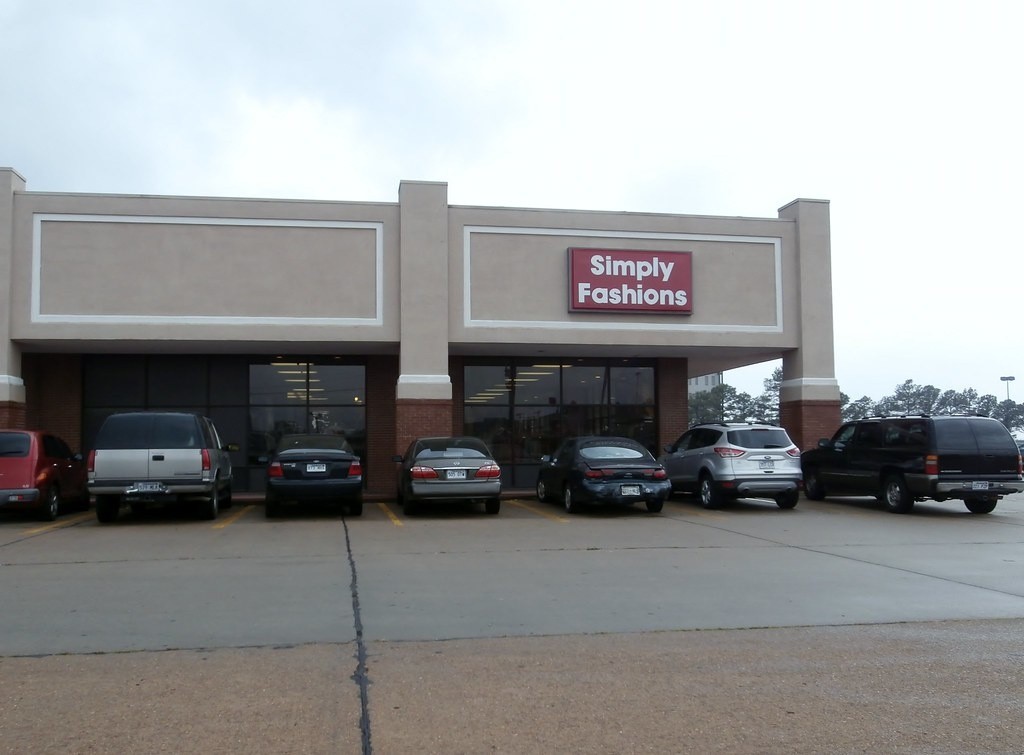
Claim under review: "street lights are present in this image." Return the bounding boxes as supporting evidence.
[1001,376,1016,399]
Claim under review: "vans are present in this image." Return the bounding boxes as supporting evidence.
[86,411,241,523]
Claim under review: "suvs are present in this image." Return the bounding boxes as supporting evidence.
[800,413,1024,515]
[659,420,803,510]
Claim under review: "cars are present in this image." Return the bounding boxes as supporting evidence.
[392,435,504,515]
[536,436,672,516]
[256,434,364,517]
[0,427,87,522]
